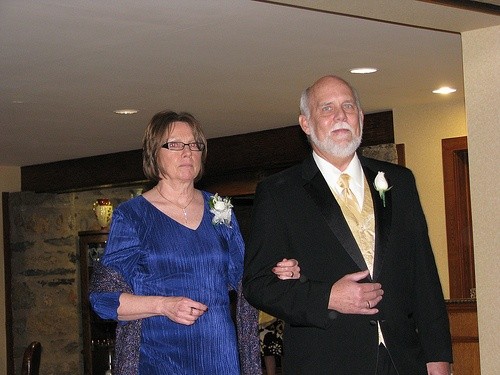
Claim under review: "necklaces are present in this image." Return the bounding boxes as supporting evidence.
[157,186,195,224]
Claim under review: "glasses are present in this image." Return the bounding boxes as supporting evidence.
[161,142,205,151]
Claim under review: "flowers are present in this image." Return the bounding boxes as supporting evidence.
[375,171,392,204]
[209,193,233,225]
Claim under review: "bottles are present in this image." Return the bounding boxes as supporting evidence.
[91,336,115,375]
[87,242,105,266]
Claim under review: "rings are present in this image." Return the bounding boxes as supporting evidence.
[291,271,294,278]
[366,301,373,310]
[189,306,193,316]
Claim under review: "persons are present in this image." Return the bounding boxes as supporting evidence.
[243,75,453,375]
[89,110,300,375]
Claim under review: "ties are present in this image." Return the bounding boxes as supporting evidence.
[337,173,361,213]
